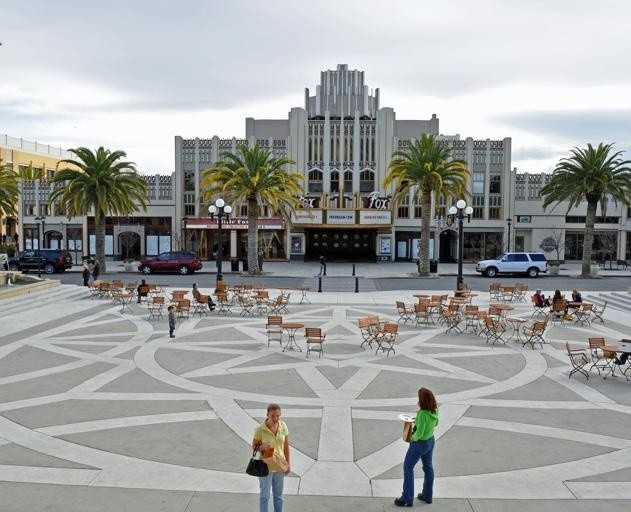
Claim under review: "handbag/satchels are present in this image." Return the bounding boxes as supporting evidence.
[246,458,269,477]
[403,422,412,442]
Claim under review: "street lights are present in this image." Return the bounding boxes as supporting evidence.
[447,199,474,299]
[34,214,47,280]
[208,199,232,294]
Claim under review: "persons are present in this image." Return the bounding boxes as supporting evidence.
[395,387,439,507]
[253,403,290,512]
[83,260,100,285]
[193,283,216,311]
[615,352,631,364]
[137,279,150,304]
[168,307,175,338]
[534,289,582,318]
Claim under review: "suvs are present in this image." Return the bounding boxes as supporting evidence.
[139,251,202,275]
[4,249,73,275]
[476,252,548,277]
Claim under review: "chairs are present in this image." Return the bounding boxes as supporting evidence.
[358,283,608,357]
[87,279,291,322]
[304,328,326,358]
[589,338,614,375]
[266,316,283,347]
[566,341,592,380]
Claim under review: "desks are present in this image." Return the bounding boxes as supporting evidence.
[600,346,631,381]
[280,323,304,353]
[296,287,312,304]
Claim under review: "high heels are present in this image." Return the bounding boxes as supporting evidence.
[418,494,432,503]
[395,498,412,506]
